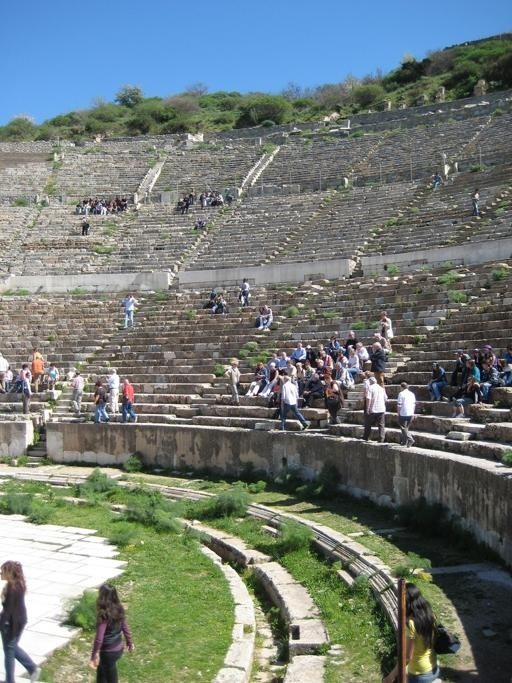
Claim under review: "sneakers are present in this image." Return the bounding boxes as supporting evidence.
[360,437,415,448]
[94,411,137,423]
[31,667,41,683]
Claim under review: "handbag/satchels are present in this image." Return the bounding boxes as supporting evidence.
[434,624,460,653]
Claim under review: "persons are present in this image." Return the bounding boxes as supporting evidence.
[241,278,250,306]
[426,345,512,418]
[89,583,134,683]
[203,292,226,314]
[397,382,416,448]
[362,376,388,442]
[93,381,110,423]
[177,189,224,215]
[76,196,128,216]
[82,219,89,236]
[106,369,120,414]
[120,379,137,423]
[381,583,439,682]
[71,372,84,413]
[278,376,308,430]
[257,305,273,331]
[194,218,205,230]
[0,561,42,683]
[322,374,345,424]
[121,294,138,329]
[471,189,480,215]
[433,173,442,188]
[0,347,60,413]
[224,310,395,406]
